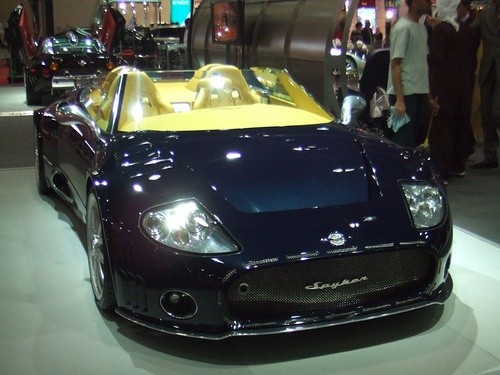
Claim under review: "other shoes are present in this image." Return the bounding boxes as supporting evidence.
[452,169,465,176]
[471,160,499,168]
[442,179,448,185]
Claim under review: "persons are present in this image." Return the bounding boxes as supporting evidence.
[350,20,383,48]
[425,0,500,185]
[386,0,439,148]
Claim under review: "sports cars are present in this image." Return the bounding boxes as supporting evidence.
[32,61,455,342]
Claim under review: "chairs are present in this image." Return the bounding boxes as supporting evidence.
[100,70,175,122]
[191,65,259,111]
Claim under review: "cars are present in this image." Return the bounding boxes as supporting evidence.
[22,26,132,106]
[150,24,186,45]
